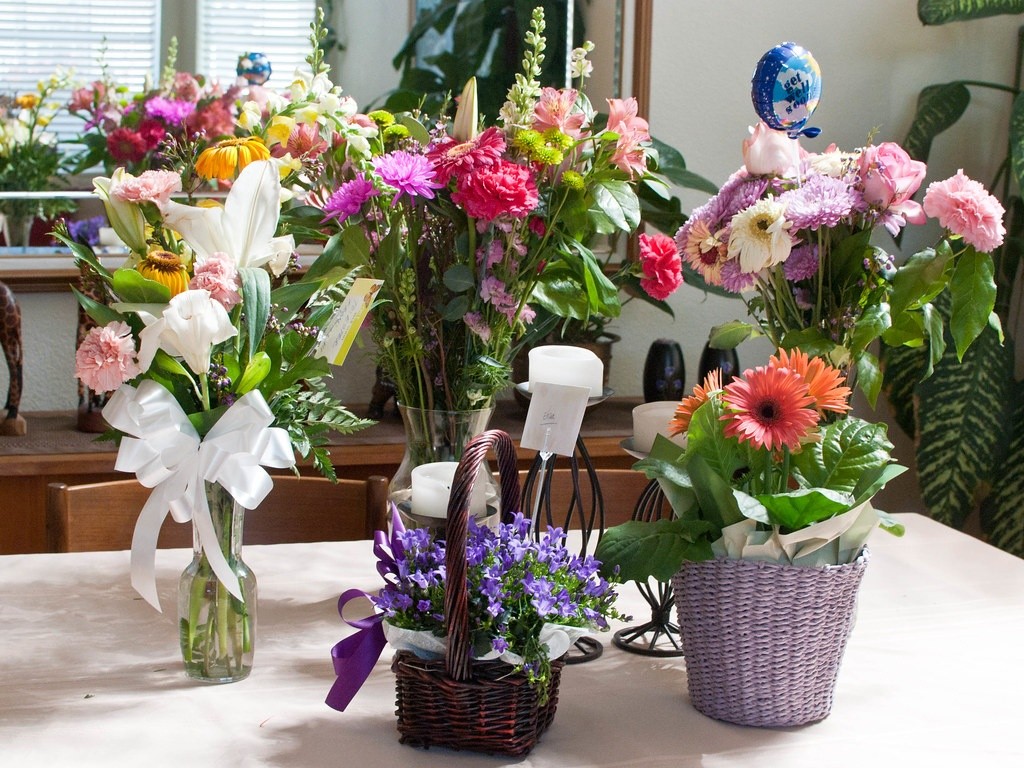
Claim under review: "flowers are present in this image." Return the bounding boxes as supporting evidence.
[0,8,1008,708]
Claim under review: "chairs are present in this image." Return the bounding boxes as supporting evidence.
[47,475,391,555]
[494,469,674,533]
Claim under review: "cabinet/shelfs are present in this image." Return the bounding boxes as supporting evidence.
[0,398,693,554]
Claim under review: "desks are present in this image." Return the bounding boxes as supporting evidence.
[0,513,1024,768]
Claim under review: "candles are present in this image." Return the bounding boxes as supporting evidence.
[632,401,689,454]
[411,461,487,519]
[529,345,604,397]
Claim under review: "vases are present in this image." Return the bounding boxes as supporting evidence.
[513,330,621,408]
[179,482,258,683]
[1,212,35,245]
[674,548,870,725]
[386,400,498,536]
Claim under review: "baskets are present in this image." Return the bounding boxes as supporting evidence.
[392,429,567,759]
[669,544,871,727]
